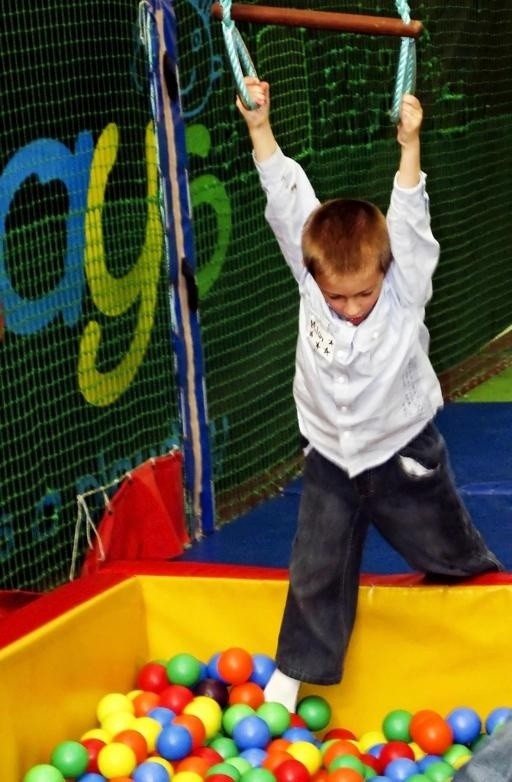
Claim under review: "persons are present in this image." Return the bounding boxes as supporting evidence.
[235,76,507,714]
